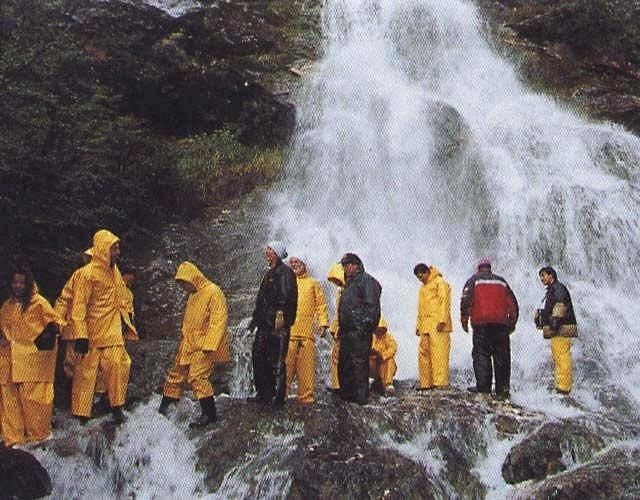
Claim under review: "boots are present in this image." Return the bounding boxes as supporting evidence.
[199,396,216,425]
[158,397,179,418]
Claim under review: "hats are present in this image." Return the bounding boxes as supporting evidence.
[267,240,288,260]
[478,256,491,265]
[342,253,361,264]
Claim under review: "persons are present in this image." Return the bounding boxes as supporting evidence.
[327,261,345,391]
[369,317,397,398]
[276,254,329,403]
[414,264,453,389]
[158,261,230,430]
[461,259,519,401]
[1,229,141,449]
[535,268,579,394]
[332,254,382,406]
[244,242,298,406]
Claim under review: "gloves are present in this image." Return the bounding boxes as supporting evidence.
[462,322,468,331]
[74,339,89,353]
[274,310,284,332]
